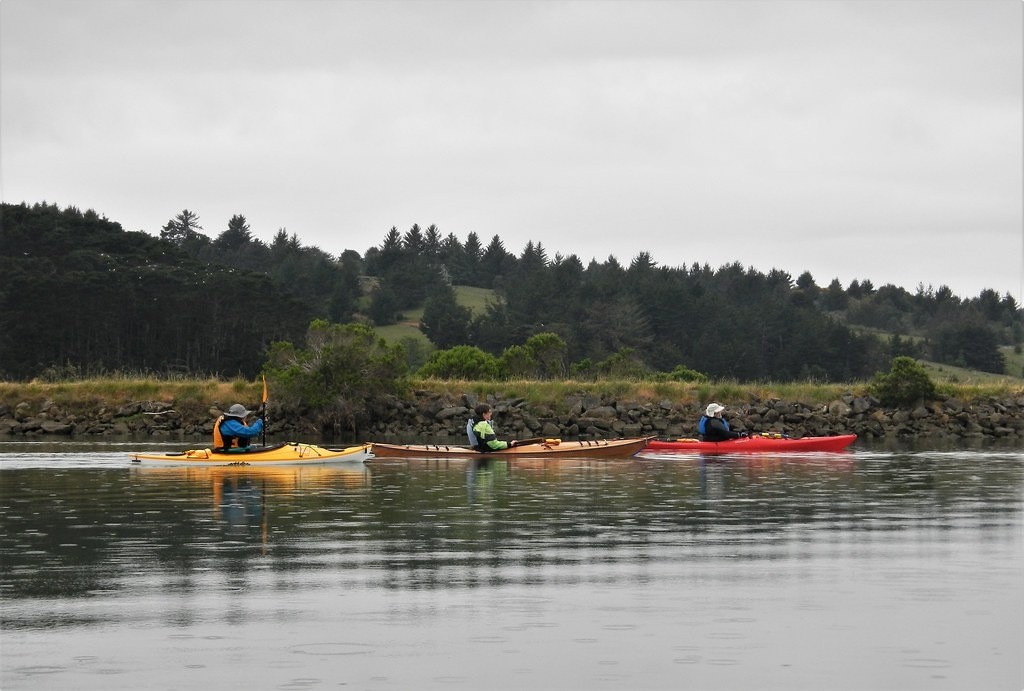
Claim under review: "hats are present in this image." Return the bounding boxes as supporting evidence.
[223,403,251,418]
[475,404,490,415]
[706,403,725,417]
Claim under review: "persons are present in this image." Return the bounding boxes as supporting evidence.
[216,404,265,454]
[698,403,749,442]
[467,404,518,452]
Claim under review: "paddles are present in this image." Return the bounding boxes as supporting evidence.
[261,374,269,446]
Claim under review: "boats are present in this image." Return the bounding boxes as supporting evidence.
[364,433,660,460]
[126,442,374,467]
[644,434,861,455]
